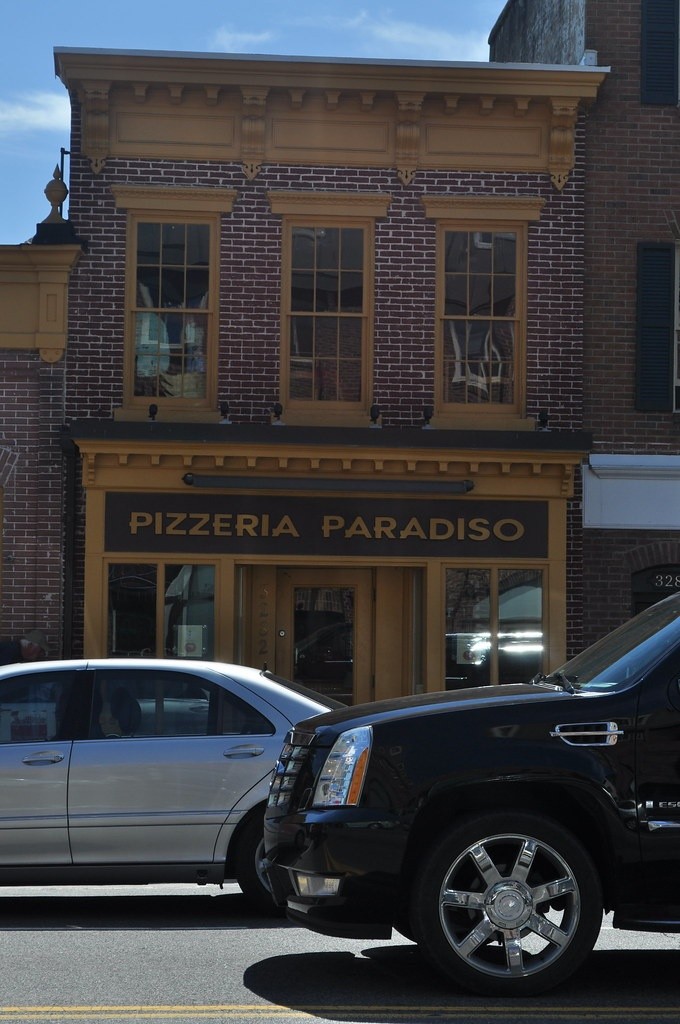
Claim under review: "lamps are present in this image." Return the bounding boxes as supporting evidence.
[369,406,382,428]
[273,403,286,425]
[422,407,433,429]
[220,401,232,424]
[538,411,552,432]
[148,404,158,420]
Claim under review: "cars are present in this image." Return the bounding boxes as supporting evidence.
[293,620,352,681]
[0,658,350,922]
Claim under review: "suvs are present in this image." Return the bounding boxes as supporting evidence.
[253,592,680,1000]
[445,629,544,691]
